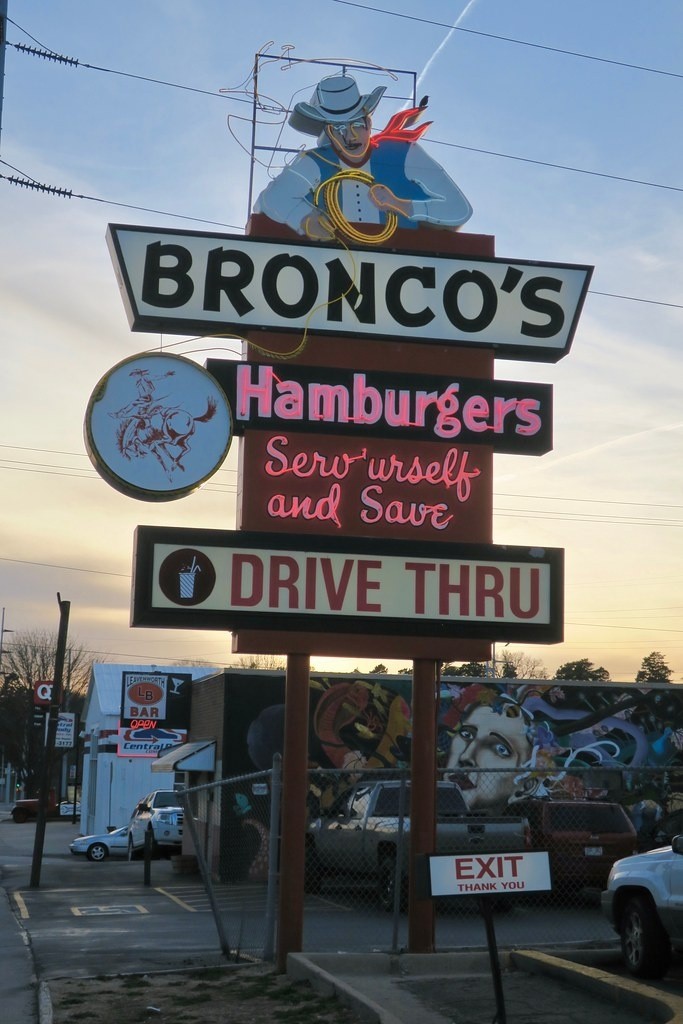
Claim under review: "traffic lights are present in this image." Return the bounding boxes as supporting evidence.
[17,777,20,787]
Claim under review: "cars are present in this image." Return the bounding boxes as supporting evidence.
[70,825,128,861]
[601,836,683,973]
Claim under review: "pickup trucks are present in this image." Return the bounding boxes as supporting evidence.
[307,779,531,913]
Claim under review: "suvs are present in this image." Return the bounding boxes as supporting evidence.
[486,797,640,899]
[127,789,184,861]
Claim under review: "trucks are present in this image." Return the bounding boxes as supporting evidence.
[10,789,81,823]
